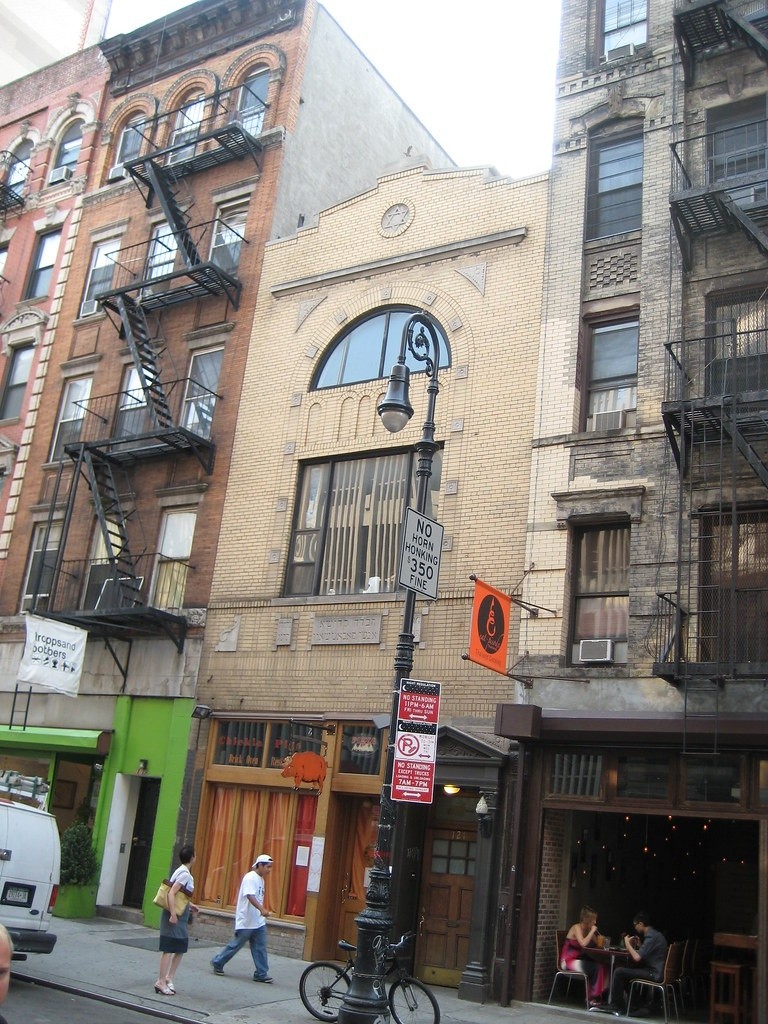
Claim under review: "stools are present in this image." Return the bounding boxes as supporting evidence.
[708,960,757,1024]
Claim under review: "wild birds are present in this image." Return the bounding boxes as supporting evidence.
[403,145,412,157]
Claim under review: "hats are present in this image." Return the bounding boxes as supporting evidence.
[252,854,273,868]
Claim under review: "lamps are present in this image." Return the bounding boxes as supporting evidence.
[372,714,391,730]
[475,796,491,840]
[108,161,130,179]
[444,785,461,793]
[135,761,147,776]
[190,705,210,720]
[79,298,102,317]
[48,166,71,184]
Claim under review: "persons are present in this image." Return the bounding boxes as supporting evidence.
[153,845,200,996]
[209,854,275,984]
[0,923,14,1024]
[609,912,668,1011]
[559,904,606,1007]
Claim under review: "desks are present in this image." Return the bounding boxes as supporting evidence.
[710,928,757,1024]
[580,944,631,1009]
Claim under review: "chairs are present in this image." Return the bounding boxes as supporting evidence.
[626,934,713,1024]
[543,929,603,1012]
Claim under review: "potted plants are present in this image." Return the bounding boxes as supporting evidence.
[51,822,99,922]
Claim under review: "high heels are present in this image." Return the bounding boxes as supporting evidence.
[154,977,176,995]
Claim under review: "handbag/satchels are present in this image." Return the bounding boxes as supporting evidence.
[153,879,192,917]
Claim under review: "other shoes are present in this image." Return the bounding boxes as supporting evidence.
[210,957,224,975]
[254,973,274,983]
[585,998,601,1007]
[629,1007,651,1018]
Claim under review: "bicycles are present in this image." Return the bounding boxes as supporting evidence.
[299,928,440,1024]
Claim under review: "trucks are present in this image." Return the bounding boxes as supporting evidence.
[0,801,62,961]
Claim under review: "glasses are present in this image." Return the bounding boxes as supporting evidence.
[632,922,638,927]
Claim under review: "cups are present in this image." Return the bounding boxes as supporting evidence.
[597,935,603,948]
[603,937,611,950]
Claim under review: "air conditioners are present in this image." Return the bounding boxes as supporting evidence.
[606,43,637,61]
[578,639,617,664]
[592,411,626,429]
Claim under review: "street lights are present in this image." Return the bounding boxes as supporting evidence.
[336,312,447,1024]
